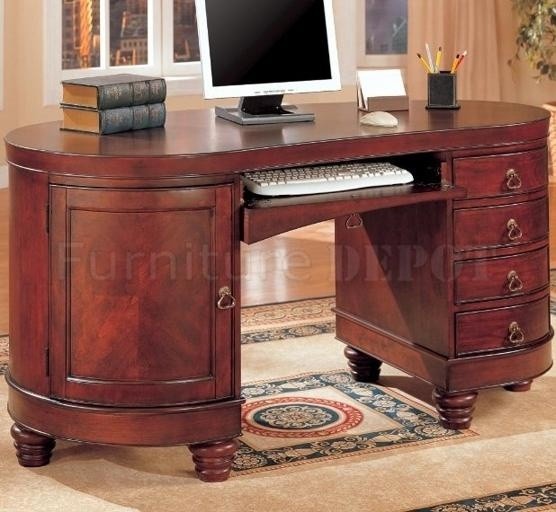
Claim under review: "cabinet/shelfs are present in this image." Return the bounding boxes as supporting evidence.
[3,100,556,481]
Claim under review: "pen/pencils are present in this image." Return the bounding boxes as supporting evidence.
[417,53,431,73]
[425,41,434,73]
[451,54,460,74]
[452,50,468,74]
[436,46,442,69]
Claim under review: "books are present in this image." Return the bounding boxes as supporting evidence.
[61,73,167,109]
[59,104,166,134]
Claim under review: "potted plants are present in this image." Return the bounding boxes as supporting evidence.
[507,1,556,185]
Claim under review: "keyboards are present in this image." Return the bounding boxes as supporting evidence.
[240,161,416,196]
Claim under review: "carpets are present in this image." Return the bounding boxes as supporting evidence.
[1,268,556,511]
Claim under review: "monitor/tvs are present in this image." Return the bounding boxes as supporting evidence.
[359,111,400,126]
[193,0,341,125]
